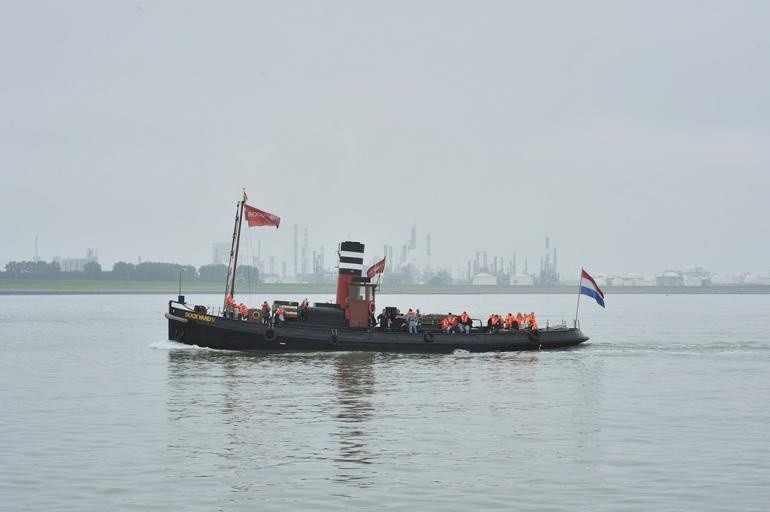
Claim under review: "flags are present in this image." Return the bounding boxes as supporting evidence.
[244,203,280,228]
[580,270,606,307]
[367,257,385,279]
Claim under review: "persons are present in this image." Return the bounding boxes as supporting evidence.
[275,305,285,322]
[376,307,539,335]
[300,297,309,321]
[262,301,271,324]
[226,295,249,321]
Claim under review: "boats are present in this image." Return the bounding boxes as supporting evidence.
[165,202,591,353]
